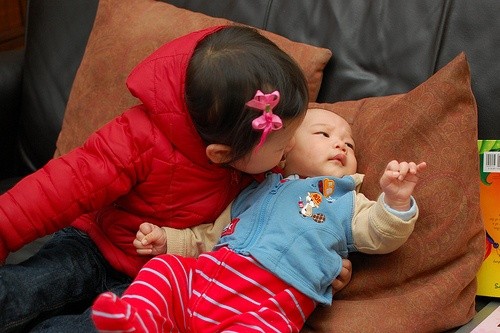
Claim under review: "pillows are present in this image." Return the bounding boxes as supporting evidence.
[48,0,332,161]
[301,49,484,333]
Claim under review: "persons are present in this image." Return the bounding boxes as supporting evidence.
[92,107,427,333]
[0,25,352,333]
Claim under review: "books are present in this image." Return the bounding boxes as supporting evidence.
[474,140,500,299]
